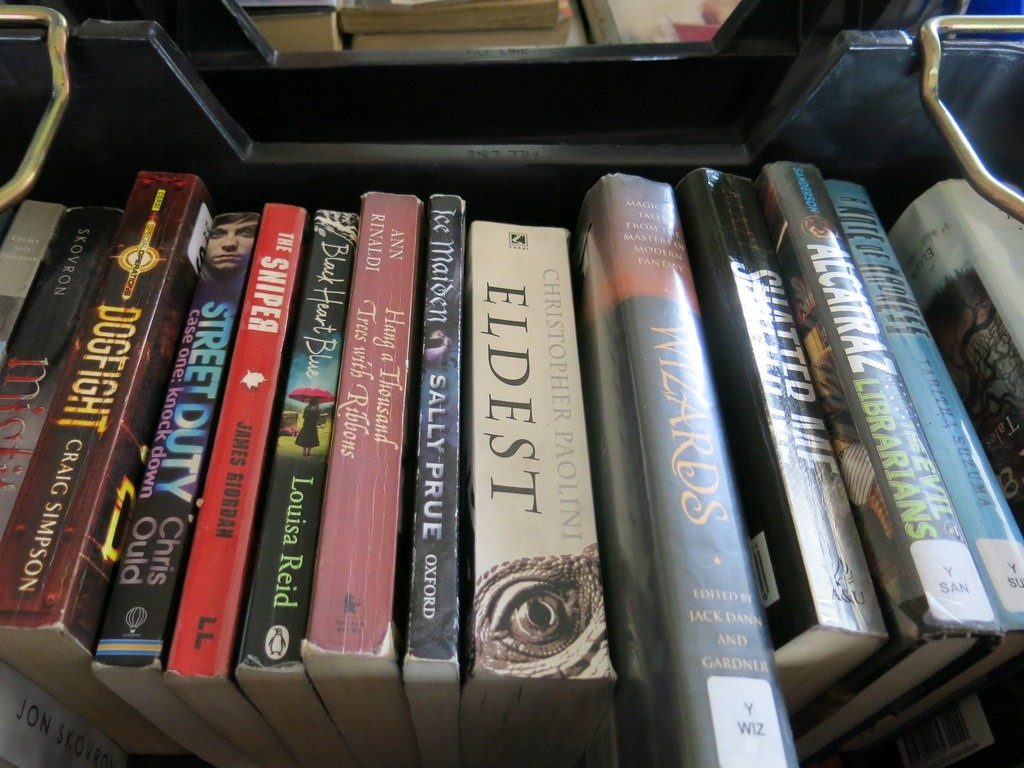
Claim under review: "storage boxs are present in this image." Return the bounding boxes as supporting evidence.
[0,0,1024,236]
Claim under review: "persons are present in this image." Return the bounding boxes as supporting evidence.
[199,212,260,299]
[294,397,321,455]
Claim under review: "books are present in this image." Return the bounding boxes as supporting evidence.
[0,0,1024,768]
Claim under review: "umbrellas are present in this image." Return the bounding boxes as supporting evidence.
[288,387,335,404]
